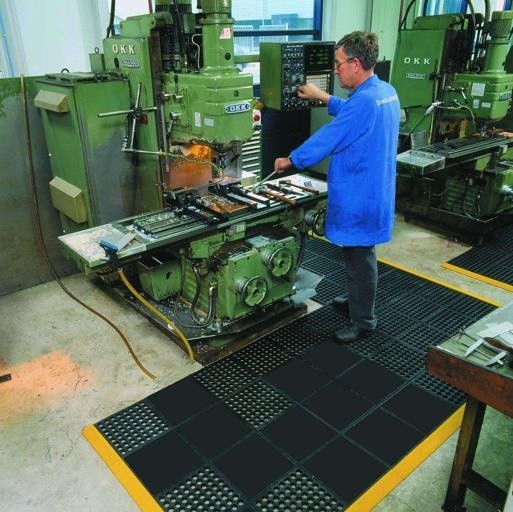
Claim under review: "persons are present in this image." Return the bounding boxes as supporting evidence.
[274,31,401,344]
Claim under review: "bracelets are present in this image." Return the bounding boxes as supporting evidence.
[319,91,327,100]
[288,155,295,167]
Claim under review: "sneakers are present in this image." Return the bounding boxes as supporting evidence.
[332,296,350,313]
[333,323,376,343]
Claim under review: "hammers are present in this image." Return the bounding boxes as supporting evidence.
[254,185,296,206]
[279,180,319,195]
